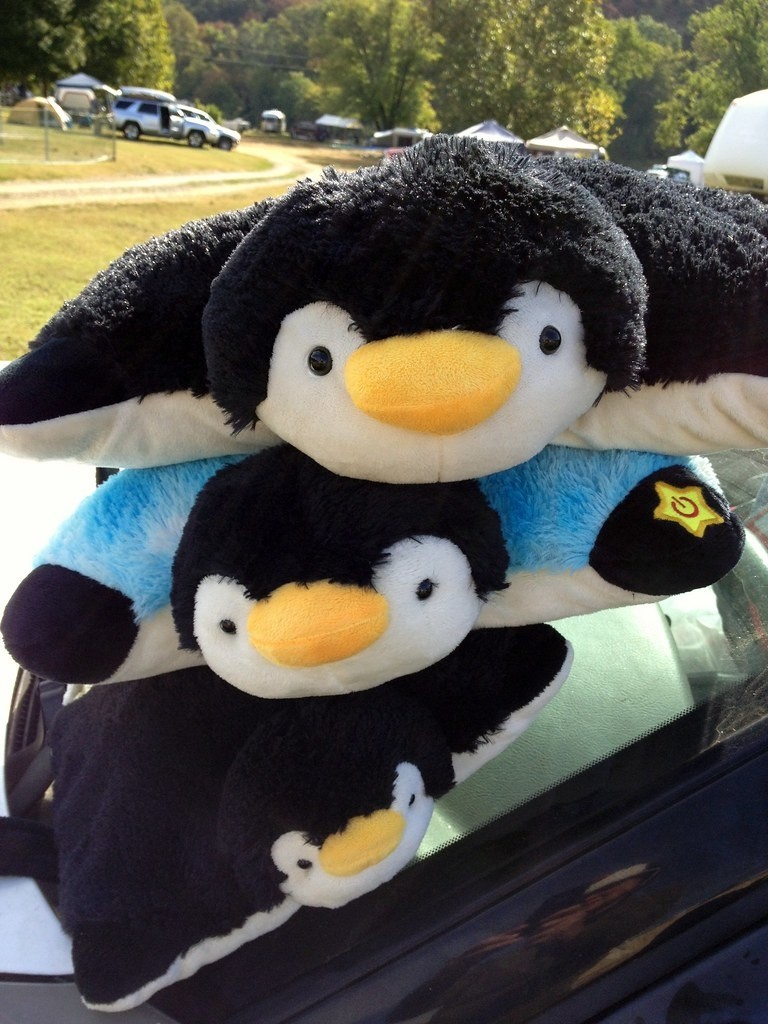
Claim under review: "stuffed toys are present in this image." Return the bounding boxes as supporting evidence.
[0,444,750,701]
[45,623,576,1013]
[0,134,768,487]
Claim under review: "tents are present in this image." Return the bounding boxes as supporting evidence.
[56,72,105,90]
[455,118,523,147]
[525,124,599,153]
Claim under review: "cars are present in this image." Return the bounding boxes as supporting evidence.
[178,105,241,151]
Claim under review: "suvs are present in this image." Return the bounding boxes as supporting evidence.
[111,97,220,148]
[646,164,668,179]
